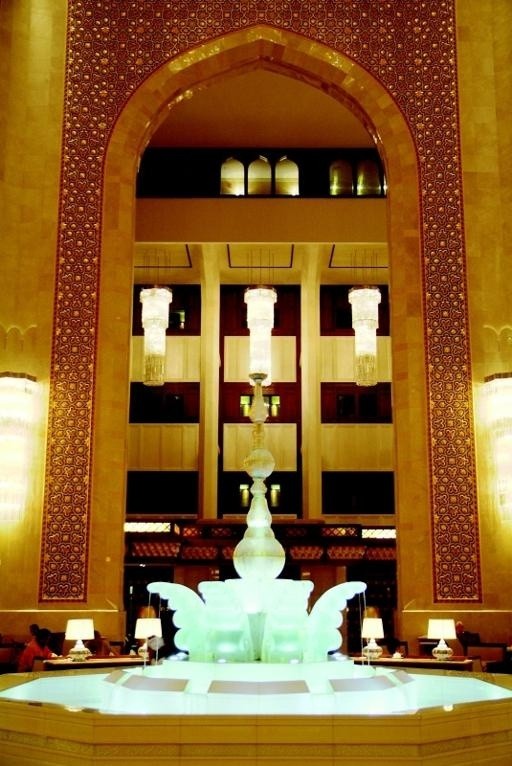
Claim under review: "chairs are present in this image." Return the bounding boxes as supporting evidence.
[450,645,508,673]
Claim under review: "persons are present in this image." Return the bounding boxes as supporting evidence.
[82,629,111,656]
[455,620,477,644]
[15,628,60,672]
[24,623,43,646]
[377,635,402,659]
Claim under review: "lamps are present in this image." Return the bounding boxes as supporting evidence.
[243,248,280,389]
[428,618,458,661]
[138,248,173,387]
[478,369,512,528]
[65,618,96,662]
[1,369,43,533]
[348,247,384,388]
[361,617,386,659]
[134,617,162,659]
